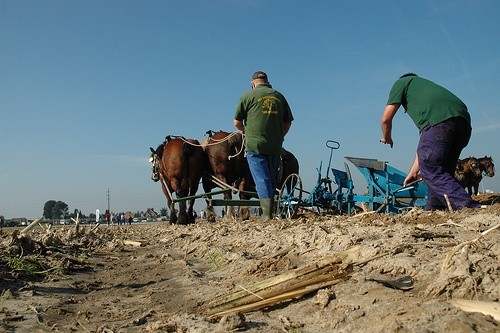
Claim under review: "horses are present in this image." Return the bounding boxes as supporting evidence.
[149,138,205,225]
[202,131,245,224]
[233,147,300,221]
[418,156,481,197]
[465,155,495,196]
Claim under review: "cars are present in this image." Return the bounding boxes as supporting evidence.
[0,215,167,227]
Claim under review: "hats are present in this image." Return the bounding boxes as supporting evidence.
[253,71,268,79]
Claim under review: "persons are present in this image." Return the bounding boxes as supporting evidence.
[254,208,258,216]
[129,215,133,225]
[379,72,482,210]
[106,211,126,225]
[233,71,295,222]
[221,207,226,218]
[193,210,206,219]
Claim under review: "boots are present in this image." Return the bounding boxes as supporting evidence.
[257,199,271,221]
